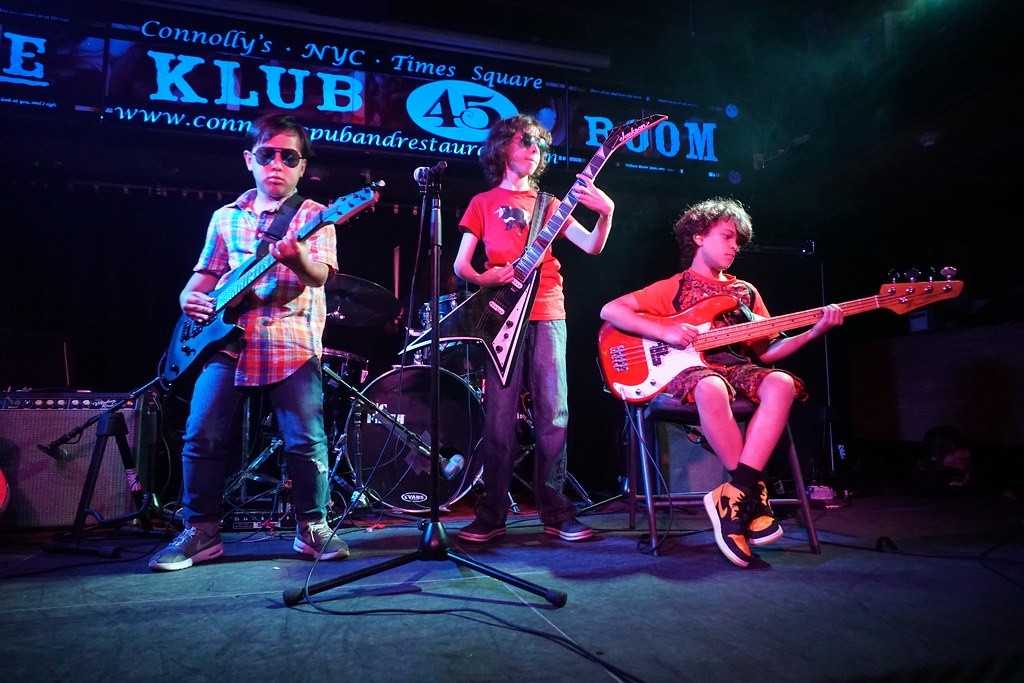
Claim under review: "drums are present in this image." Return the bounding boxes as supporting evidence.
[421,290,477,364]
[342,365,489,514]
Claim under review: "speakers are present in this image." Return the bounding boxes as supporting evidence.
[715,250,835,495]
[0,389,159,534]
[637,417,744,496]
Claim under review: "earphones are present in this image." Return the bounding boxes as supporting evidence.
[696,235,704,241]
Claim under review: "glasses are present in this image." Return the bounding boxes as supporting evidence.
[504,133,550,151]
[252,147,304,168]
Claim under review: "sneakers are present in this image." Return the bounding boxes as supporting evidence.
[703,482,752,568]
[291,518,349,560]
[544,517,593,541]
[148,522,224,571]
[745,480,783,546]
[459,517,507,542]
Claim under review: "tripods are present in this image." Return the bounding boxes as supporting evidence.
[220,162,567,607]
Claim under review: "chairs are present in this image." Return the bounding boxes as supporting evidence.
[595,356,821,558]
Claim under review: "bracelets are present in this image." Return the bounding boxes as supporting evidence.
[601,213,612,218]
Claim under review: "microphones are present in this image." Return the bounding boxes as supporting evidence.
[442,454,465,481]
[413,167,431,181]
[37,443,68,461]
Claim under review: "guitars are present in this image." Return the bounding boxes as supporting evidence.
[598,266,962,402]
[320,348,368,391]
[399,114,669,386]
[164,184,381,385]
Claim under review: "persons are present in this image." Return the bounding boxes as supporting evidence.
[454,115,615,542]
[149,112,349,571]
[601,196,844,567]
